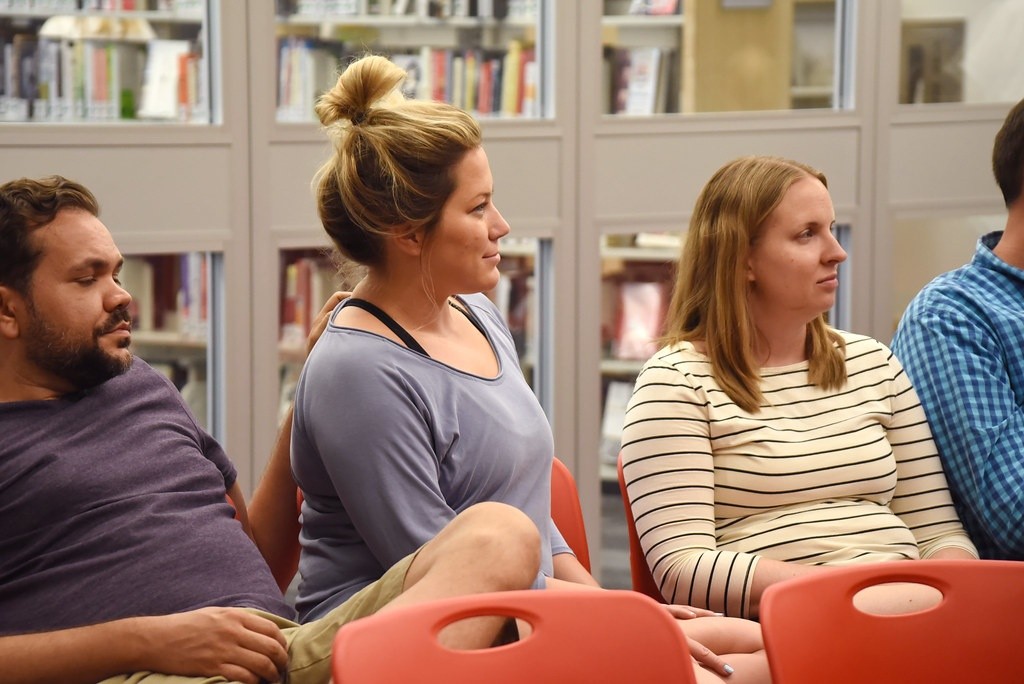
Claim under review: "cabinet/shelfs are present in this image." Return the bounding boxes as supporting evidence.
[0,0,796,485]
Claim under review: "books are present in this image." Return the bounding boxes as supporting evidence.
[483,238,538,387]
[276,255,345,431]
[600,2,681,116]
[272,2,543,123]
[597,231,688,479]
[2,0,215,126]
[114,248,209,433]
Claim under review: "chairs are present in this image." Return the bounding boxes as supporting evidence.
[617,452,667,605]
[296,457,592,575]
[758,559,1024,684]
[328,588,699,684]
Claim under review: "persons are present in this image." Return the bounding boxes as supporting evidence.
[1,176,544,683]
[621,154,980,615]
[887,99,1023,563]
[287,56,772,684]
